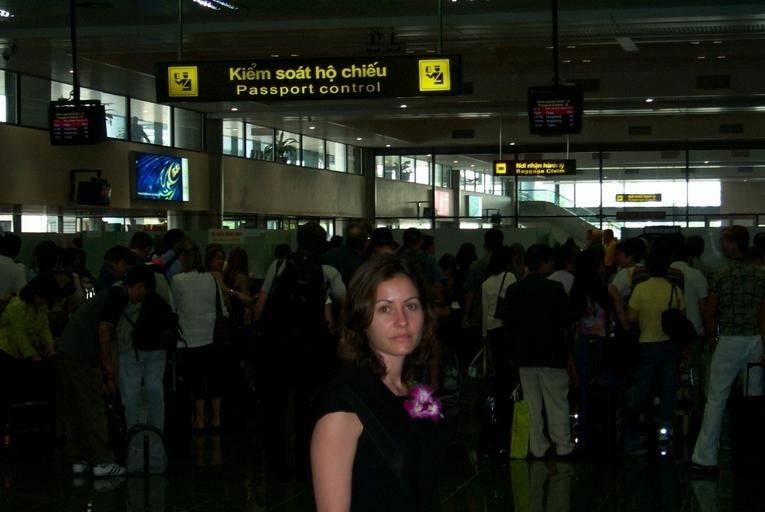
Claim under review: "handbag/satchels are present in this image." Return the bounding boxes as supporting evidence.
[213,276,233,346]
[455,344,503,414]
[492,271,508,321]
[660,286,685,336]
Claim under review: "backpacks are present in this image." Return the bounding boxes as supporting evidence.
[123,423,168,478]
[127,293,182,351]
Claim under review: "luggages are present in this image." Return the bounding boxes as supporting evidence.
[2,359,56,467]
[730,361,765,511]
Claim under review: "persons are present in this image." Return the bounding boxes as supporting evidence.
[0,211,764,511]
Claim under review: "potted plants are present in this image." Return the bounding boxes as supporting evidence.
[393,159,412,181]
[264,130,299,164]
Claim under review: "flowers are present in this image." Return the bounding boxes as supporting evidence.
[400,381,446,420]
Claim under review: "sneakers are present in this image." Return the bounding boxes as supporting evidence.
[72,460,90,474]
[623,447,647,457]
[92,461,126,478]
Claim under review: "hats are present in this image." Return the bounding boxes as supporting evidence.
[370,228,396,245]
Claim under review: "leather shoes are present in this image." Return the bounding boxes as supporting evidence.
[689,466,719,480]
